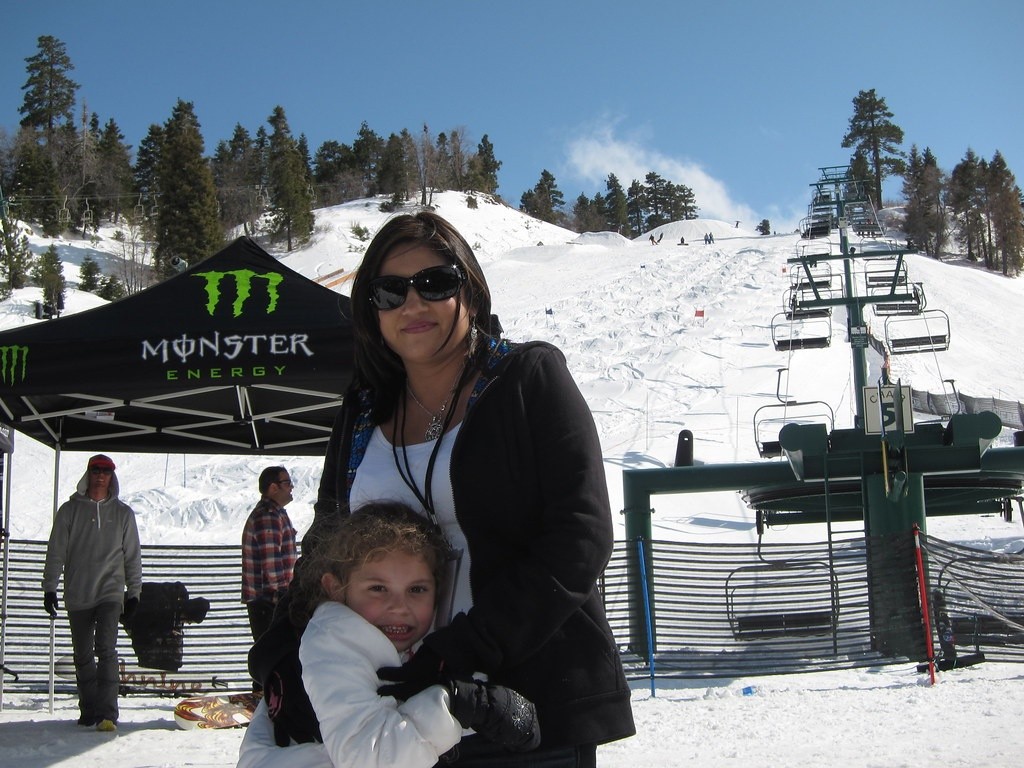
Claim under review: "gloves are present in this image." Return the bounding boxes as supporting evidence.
[448,674,542,754]
[125,597,138,624]
[264,664,323,748]
[377,631,456,701]
[44,592,59,617]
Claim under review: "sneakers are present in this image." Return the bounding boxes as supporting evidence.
[95,714,117,732]
[78,710,96,729]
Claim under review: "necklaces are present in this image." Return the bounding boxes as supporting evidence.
[406,368,463,442]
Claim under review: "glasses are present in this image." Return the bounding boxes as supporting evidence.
[369,264,468,310]
[92,468,112,475]
[278,480,291,484]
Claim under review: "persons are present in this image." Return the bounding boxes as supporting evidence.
[704,232,714,244]
[236,503,539,768]
[857,219,876,237]
[241,467,298,694]
[681,237,684,243]
[648,232,663,245]
[248,212,636,768]
[41,454,142,731]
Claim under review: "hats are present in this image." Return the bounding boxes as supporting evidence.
[89,455,115,471]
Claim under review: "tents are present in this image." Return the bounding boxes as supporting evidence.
[1,234,503,712]
